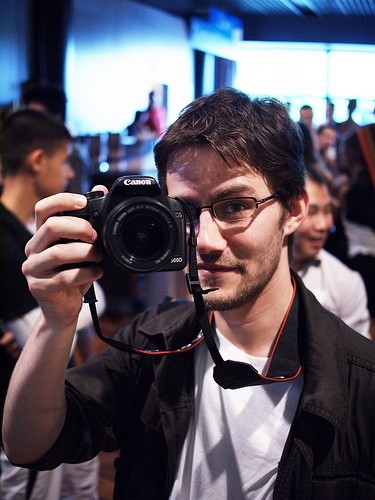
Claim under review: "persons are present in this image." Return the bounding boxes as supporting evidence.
[18,82,89,206]
[293,164,373,343]
[0,108,109,500]
[126,86,375,260]
[0,86,375,500]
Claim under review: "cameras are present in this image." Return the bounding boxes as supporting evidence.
[57,175,188,274]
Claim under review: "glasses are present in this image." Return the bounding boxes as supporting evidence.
[180,192,279,226]
